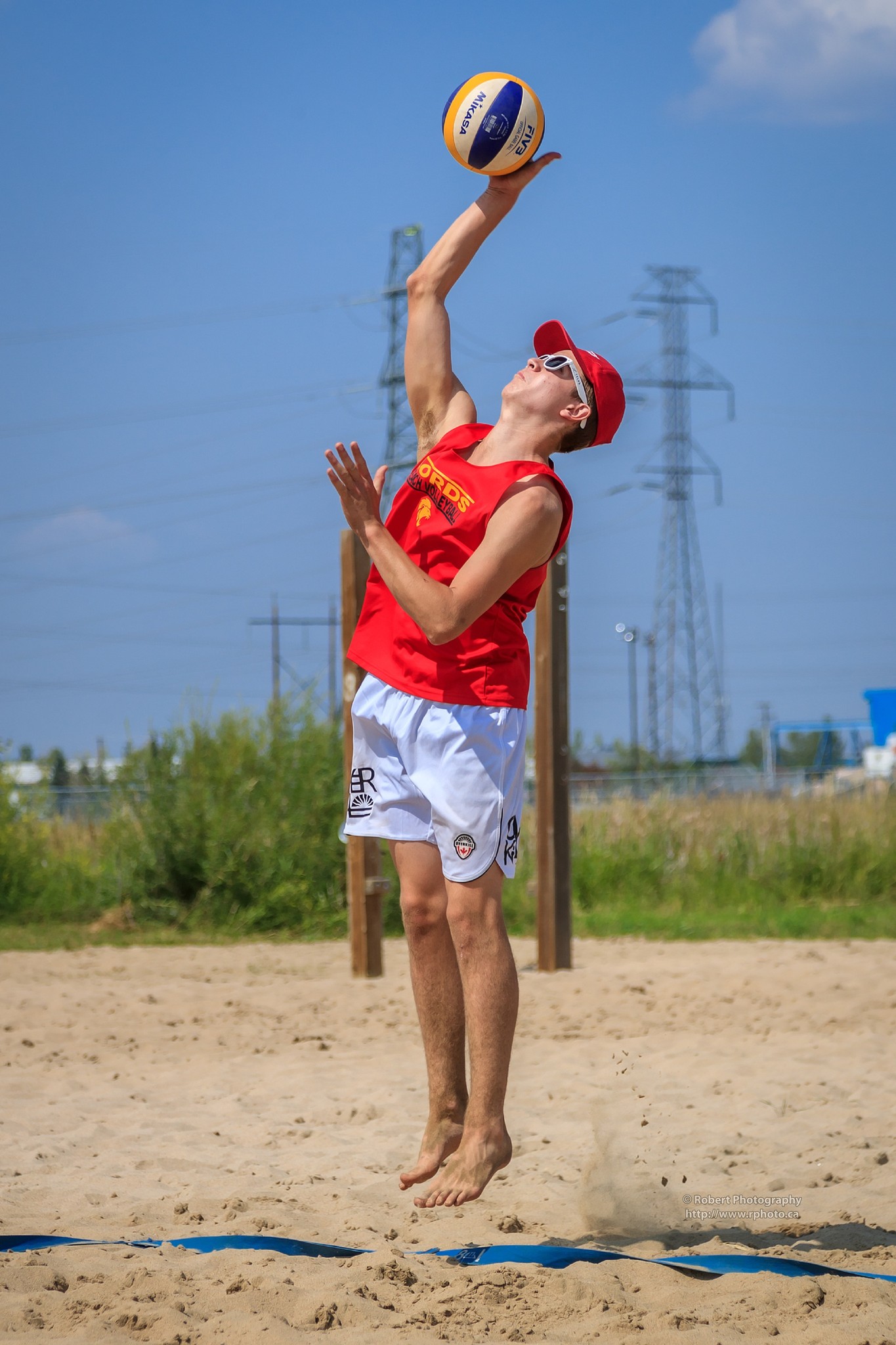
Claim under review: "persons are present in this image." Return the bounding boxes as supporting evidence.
[323,151,626,1211]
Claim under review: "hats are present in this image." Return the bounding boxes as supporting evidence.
[531,319,626,455]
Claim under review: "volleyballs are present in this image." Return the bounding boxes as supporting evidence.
[441,71,546,176]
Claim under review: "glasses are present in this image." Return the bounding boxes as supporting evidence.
[536,354,588,429]
